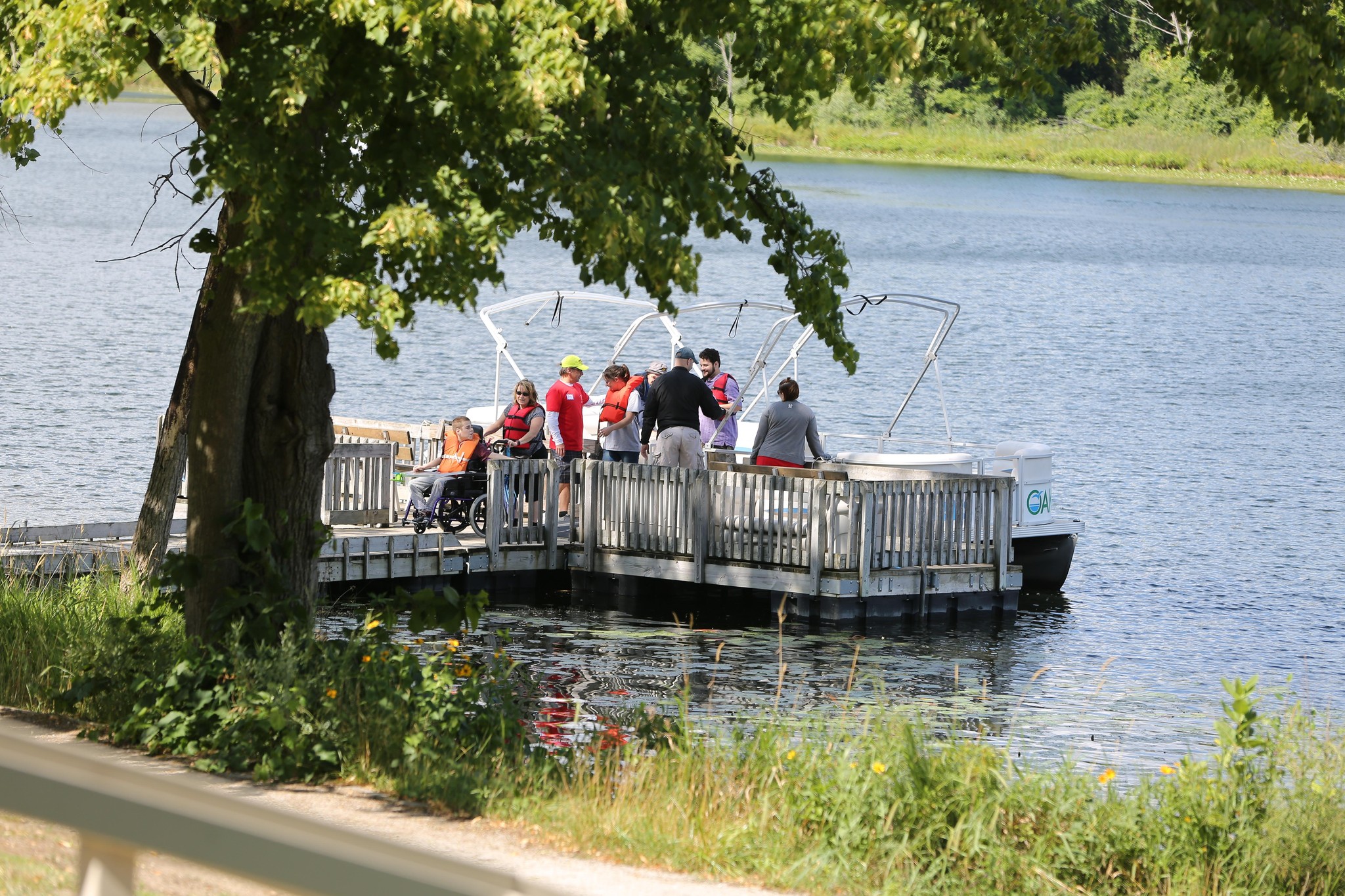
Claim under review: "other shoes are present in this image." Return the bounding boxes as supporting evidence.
[422,510,432,523]
[558,514,570,521]
[411,512,424,523]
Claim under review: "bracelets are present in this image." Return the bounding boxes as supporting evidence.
[515,440,520,446]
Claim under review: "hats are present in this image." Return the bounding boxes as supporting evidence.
[644,361,668,374]
[675,346,699,364]
[561,355,589,371]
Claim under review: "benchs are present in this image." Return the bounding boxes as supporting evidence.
[709,461,848,480]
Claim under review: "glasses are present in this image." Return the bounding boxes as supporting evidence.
[571,367,582,372]
[606,381,609,383]
[777,390,780,394]
[516,391,528,396]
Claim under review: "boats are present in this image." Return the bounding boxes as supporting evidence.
[466,290,1085,599]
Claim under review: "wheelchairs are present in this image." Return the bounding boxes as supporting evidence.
[401,424,518,538]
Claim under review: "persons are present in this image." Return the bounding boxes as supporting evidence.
[698,348,742,464]
[750,377,832,468]
[409,416,517,523]
[597,364,644,464]
[634,361,669,427]
[640,346,730,469]
[482,379,548,528]
[545,355,607,522]
